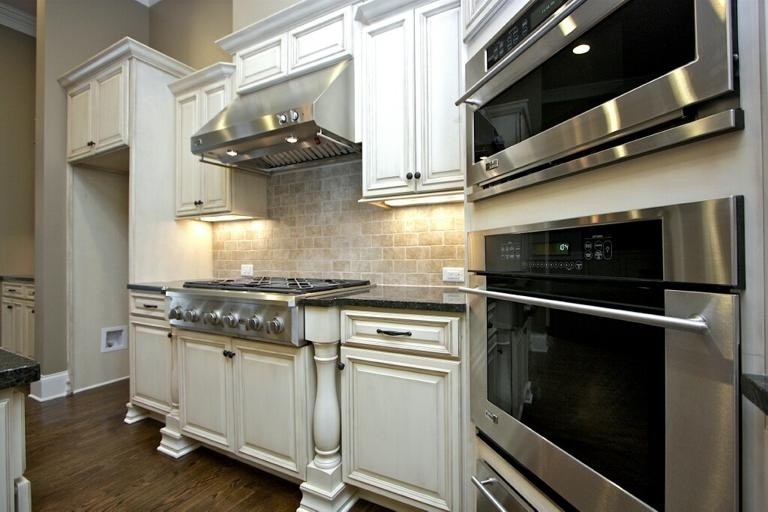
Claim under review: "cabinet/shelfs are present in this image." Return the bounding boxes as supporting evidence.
[0,282,35,360]
[56,36,201,173]
[122,286,480,511]
[168,61,272,222]
[335,287,479,512]
[176,328,340,486]
[216,0,356,96]
[124,285,174,425]
[355,2,468,210]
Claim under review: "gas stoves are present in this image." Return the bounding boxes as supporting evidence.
[151,277,370,348]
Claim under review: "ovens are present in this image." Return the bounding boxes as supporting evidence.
[453,0,745,512]
